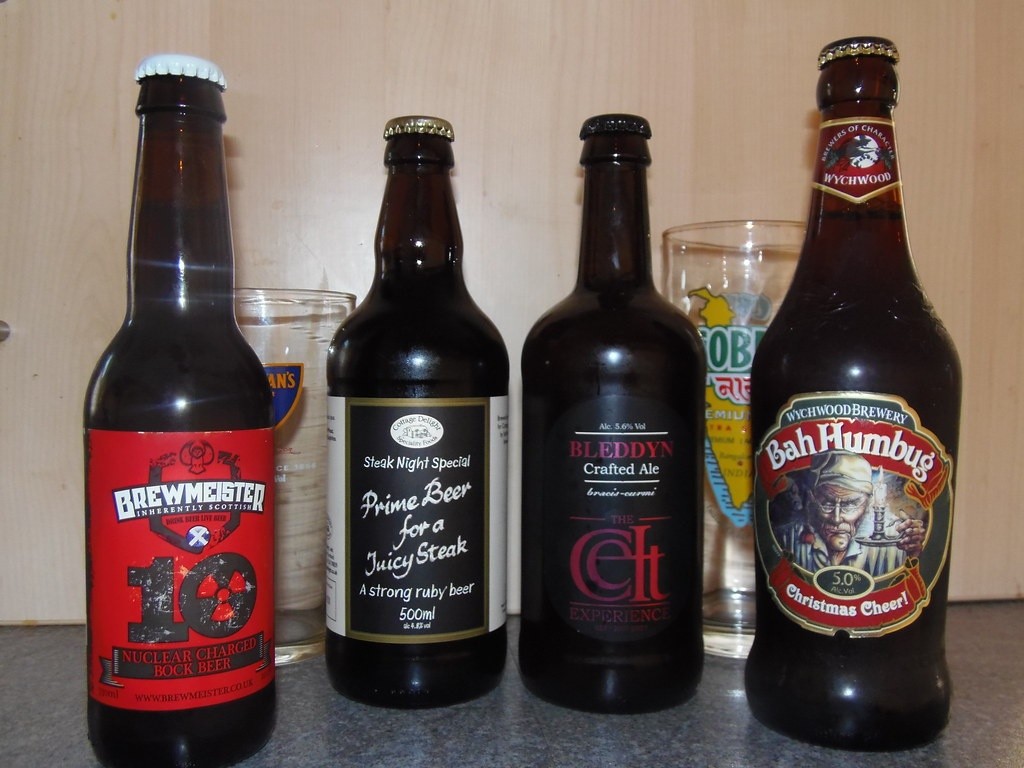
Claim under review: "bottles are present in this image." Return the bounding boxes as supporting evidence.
[81,55,277,768]
[322,114,512,708]
[738,36,965,755]
[516,113,711,715]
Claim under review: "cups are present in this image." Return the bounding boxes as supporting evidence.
[661,216,811,661]
[221,285,358,669]
